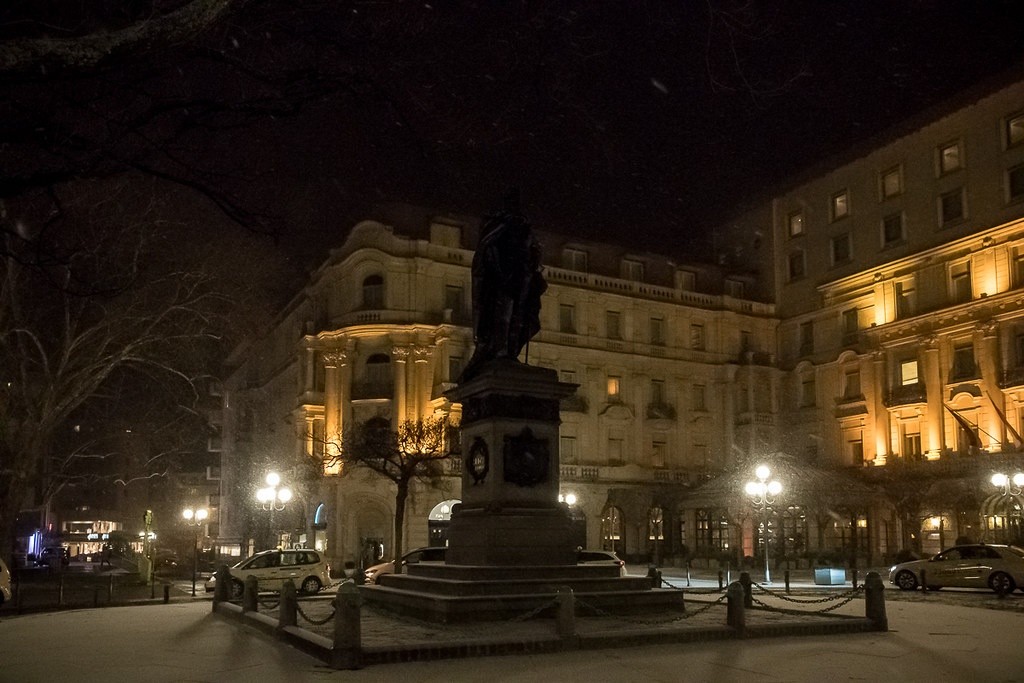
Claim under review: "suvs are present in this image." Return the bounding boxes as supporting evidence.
[208,549,329,595]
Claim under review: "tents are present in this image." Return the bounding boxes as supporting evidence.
[675,450,873,587]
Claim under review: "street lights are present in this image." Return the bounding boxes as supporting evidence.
[745,464,784,583]
[259,472,290,549]
[182,505,209,594]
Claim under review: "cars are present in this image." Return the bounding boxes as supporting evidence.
[34,547,71,564]
[889,542,1021,594]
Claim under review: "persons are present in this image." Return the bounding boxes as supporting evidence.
[100,546,112,568]
[895,540,921,567]
[455,184,549,387]
